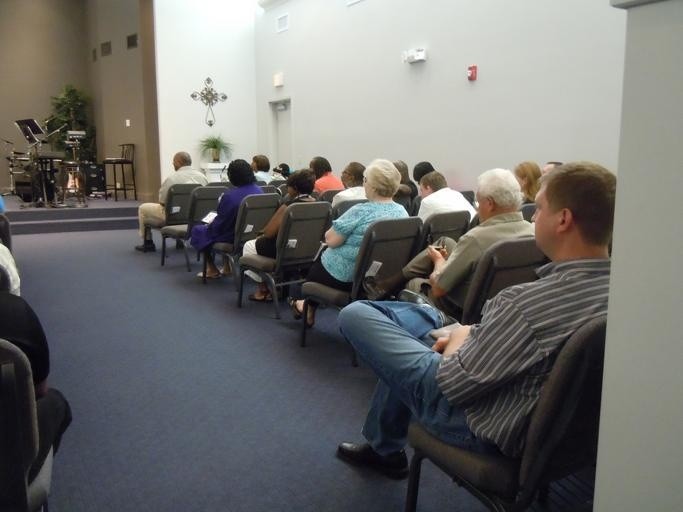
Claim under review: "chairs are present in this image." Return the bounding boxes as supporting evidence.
[60,150,89,208]
[0,212,11,252]
[0,265,10,294]
[403,315,607,510]
[0,336,53,511]
[102,144,137,201]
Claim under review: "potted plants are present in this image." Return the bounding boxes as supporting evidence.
[199,132,234,163]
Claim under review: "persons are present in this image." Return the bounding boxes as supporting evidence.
[287,157,567,320]
[0,244,22,297]
[1,288,73,484]
[333,159,619,482]
[0,194,8,215]
[134,151,317,303]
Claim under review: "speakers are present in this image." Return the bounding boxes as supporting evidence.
[85,164,105,196]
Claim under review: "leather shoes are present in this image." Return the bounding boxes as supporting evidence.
[287,296,302,319]
[247,294,270,302]
[176,240,184,249]
[196,270,220,278]
[398,288,447,327]
[364,275,388,300]
[216,267,231,276]
[134,244,155,253]
[337,439,409,476]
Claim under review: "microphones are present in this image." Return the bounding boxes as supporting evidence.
[56,123,68,134]
